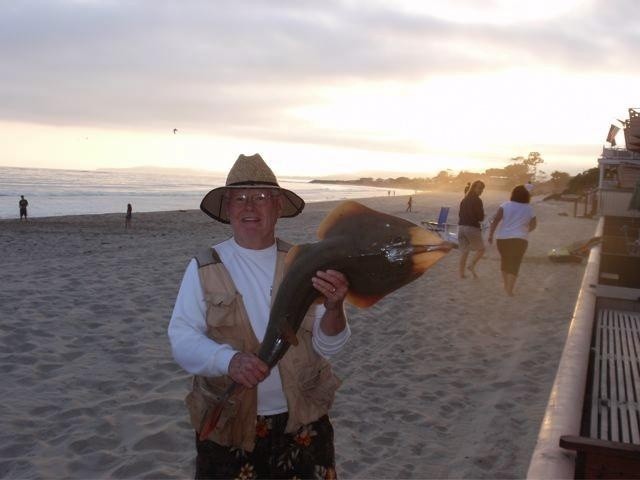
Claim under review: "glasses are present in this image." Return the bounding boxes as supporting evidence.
[229,191,278,205]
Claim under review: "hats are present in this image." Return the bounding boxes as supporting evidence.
[200,153,305,225]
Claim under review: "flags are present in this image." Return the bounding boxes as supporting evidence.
[606,124,621,143]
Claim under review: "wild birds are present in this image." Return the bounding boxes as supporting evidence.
[173,128,178,134]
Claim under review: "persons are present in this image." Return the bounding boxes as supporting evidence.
[124,204,132,228]
[464,182,471,195]
[163,151,355,480]
[405,196,412,213]
[18,194,29,220]
[487,183,537,298]
[456,180,486,280]
[525,180,535,192]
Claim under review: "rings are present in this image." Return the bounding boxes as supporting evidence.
[330,286,336,298]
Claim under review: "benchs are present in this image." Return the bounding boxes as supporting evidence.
[556,309,639,478]
